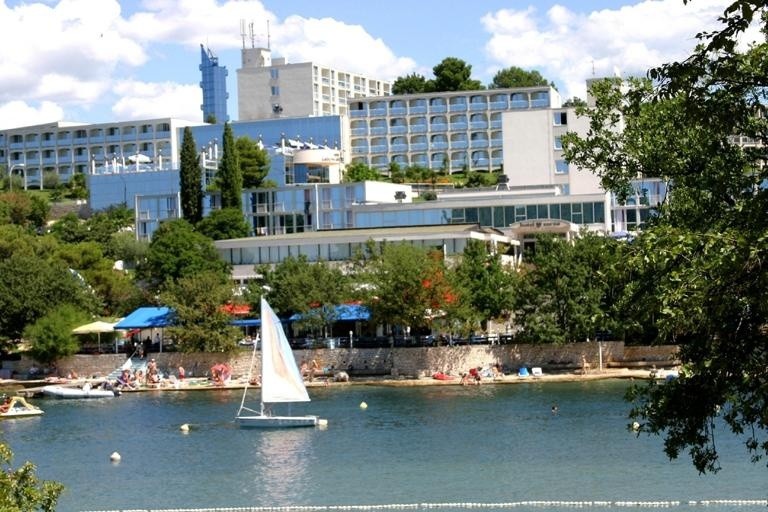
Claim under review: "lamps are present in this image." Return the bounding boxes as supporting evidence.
[115,374,184,392]
[518,367,546,380]
[1,397,34,414]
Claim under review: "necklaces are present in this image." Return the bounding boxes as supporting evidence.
[234,296,318,427]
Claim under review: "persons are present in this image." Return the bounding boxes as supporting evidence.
[309,360,318,382]
[581,355,588,376]
[28,363,78,380]
[133,342,144,360]
[245,335,252,342]
[176,364,184,381]
[301,360,308,376]
[218,368,225,384]
[123,358,160,387]
[461,373,482,386]
[329,365,335,376]
[492,363,499,377]
[112,332,160,352]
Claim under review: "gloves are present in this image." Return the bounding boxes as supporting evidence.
[0,397,44,417]
[42,382,111,398]
